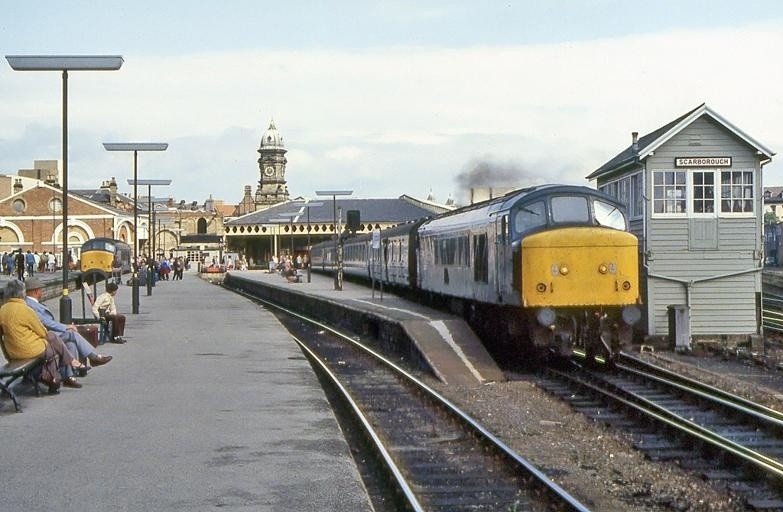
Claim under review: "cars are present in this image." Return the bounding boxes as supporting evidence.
[201,264,227,273]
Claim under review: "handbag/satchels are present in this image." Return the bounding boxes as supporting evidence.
[77,326,98,348]
[42,361,62,387]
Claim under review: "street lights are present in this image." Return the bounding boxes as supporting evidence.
[268,218,290,264]
[6,53,124,325]
[314,190,352,290]
[278,212,304,276]
[289,201,324,285]
[102,141,168,316]
[127,178,171,297]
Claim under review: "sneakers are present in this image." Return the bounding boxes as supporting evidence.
[111,337,126,344]
[90,356,112,367]
[63,376,82,388]
[72,363,91,372]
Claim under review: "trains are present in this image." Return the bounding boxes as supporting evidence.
[292,183,643,372]
[79,237,131,283]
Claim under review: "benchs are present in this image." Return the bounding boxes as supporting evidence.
[0,359,43,412]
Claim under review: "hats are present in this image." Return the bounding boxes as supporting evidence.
[25,277,47,291]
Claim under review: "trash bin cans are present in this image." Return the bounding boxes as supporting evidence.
[668,304,692,351]
[269,261,276,274]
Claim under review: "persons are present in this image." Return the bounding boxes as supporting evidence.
[127,253,189,286]
[92,283,127,344]
[2,247,74,281]
[111,255,122,284]
[0,278,93,393]
[201,254,248,271]
[271,254,308,269]
[23,281,113,389]
